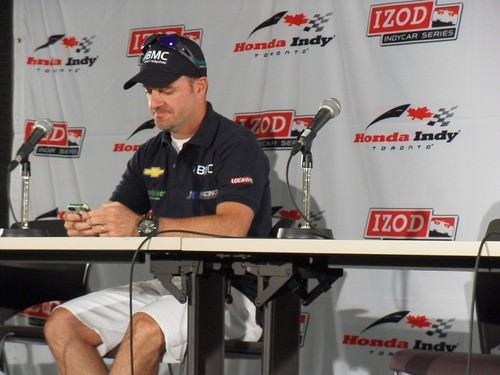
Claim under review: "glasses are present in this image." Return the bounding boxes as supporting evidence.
[141,33,204,76]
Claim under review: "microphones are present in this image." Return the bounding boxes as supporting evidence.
[7,118,53,172]
[291,98,342,156]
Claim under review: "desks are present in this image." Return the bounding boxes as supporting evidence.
[0,236,500,375]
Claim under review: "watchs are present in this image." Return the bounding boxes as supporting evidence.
[137,213,159,237]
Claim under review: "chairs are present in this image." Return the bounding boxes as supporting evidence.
[0,220,91,375]
[390,219,500,375]
[98,206,290,361]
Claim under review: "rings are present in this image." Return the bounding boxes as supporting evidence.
[101,224,104,232]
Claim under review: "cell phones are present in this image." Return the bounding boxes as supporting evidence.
[68,204,90,214]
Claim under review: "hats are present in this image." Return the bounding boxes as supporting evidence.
[123,34,207,90]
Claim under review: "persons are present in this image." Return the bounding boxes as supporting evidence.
[42,35,271,375]
